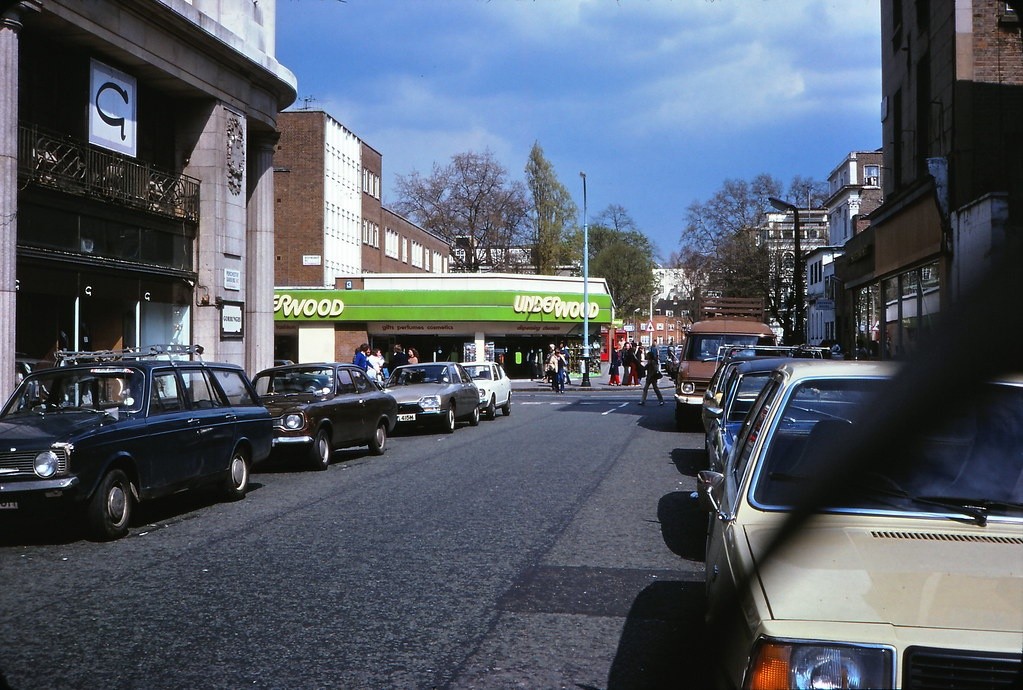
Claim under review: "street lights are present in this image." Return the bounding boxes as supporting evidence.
[767,196,804,345]
[580,171,591,387]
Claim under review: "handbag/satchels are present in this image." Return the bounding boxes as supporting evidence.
[656,371,663,379]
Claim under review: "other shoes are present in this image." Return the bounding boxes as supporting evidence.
[556,391,559,393]
[561,391,564,393]
[618,383,621,386]
[635,384,641,387]
[660,402,664,405]
[638,401,646,406]
[609,383,614,386]
[628,384,634,386]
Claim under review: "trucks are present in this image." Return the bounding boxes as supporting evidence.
[675,289,777,426]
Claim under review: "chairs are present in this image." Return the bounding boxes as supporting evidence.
[783,421,868,504]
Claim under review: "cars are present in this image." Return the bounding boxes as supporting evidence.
[459,361,512,421]
[697,361,1023,690]
[0,344,274,540]
[383,361,480,434]
[241,362,400,471]
[658,345,683,380]
[703,345,871,474]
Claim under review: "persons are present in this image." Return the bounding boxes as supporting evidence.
[525,341,571,394]
[637,352,665,406]
[817,340,867,359]
[352,342,418,384]
[608,337,676,387]
